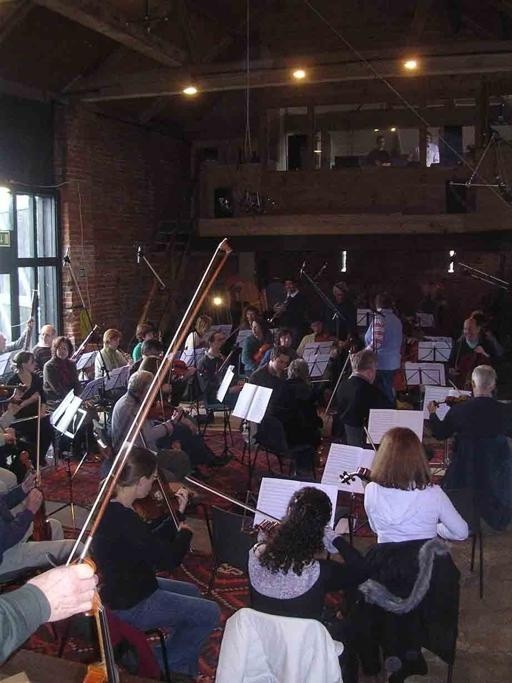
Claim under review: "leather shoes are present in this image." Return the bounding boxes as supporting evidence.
[389,658,427,683]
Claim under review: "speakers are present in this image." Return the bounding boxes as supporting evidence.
[445,179,467,213]
[214,186,234,218]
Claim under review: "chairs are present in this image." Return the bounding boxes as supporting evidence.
[1,334,512,495]
[223,608,343,683]
[441,488,486,598]
[204,504,255,597]
[85,603,173,679]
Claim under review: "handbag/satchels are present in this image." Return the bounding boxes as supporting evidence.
[182,378,200,400]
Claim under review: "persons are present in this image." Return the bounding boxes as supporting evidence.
[368,136,390,167]
[2,278,511,681]
[407,133,440,167]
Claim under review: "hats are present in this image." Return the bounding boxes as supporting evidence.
[158,448,192,483]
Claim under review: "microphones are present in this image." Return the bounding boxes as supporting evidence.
[300,260,307,279]
[136,246,142,263]
[63,248,71,267]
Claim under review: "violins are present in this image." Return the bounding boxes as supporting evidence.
[131,473,194,524]
[256,519,281,542]
[339,468,372,485]
[433,395,471,407]
[19,451,53,541]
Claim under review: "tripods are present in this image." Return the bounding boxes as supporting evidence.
[45,438,93,538]
[230,423,257,510]
[215,397,241,463]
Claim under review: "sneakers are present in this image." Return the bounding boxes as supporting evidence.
[212,454,232,466]
